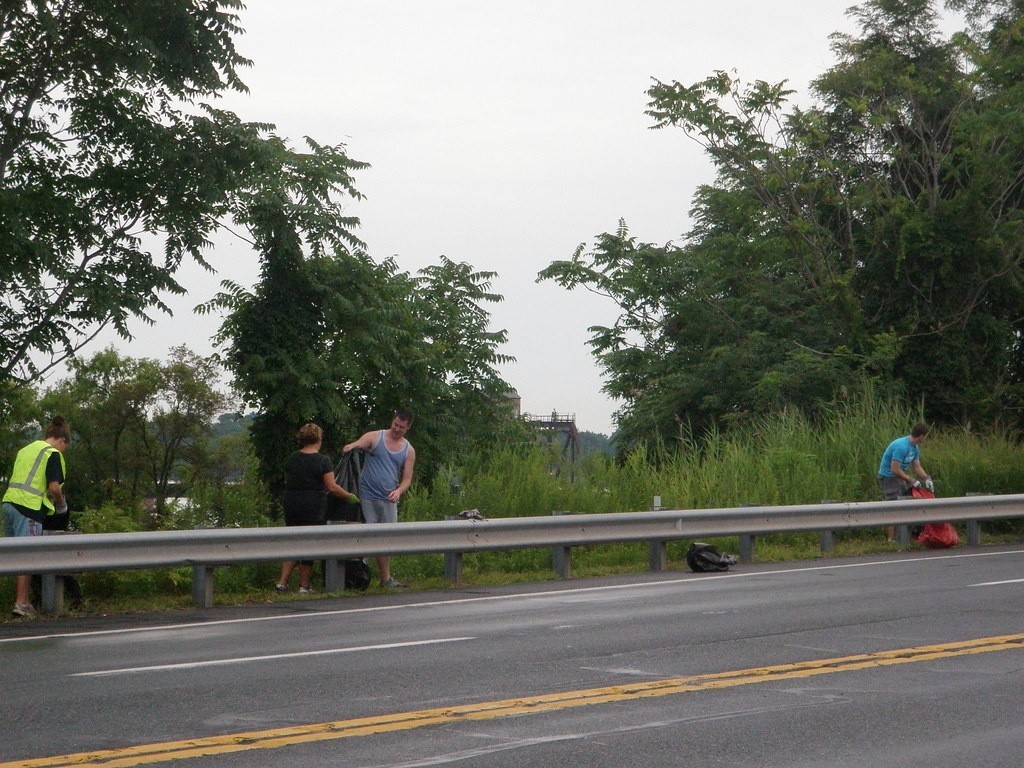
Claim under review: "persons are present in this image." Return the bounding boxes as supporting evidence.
[276,423,359,595]
[343,411,415,589]
[878,424,934,542]
[0,414,70,616]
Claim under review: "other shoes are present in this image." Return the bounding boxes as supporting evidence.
[382,577,408,589]
[299,586,318,594]
[275,583,296,594]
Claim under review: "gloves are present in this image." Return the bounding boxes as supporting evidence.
[56,496,68,515]
[347,493,361,505]
[910,478,924,489]
[924,475,936,492]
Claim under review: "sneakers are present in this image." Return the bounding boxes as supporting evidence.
[11,603,42,618]
